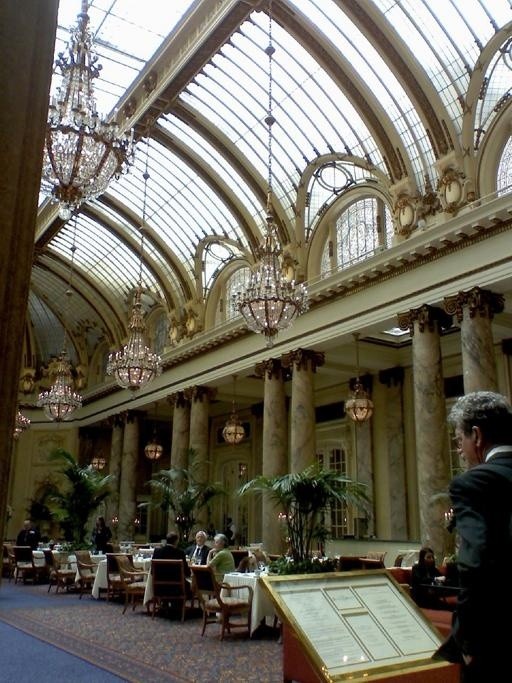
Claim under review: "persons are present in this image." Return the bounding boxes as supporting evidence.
[16,521,37,562]
[225,517,237,545]
[150,532,191,619]
[92,516,113,551]
[431,390,510,681]
[188,531,212,566]
[194,535,235,590]
[409,548,455,610]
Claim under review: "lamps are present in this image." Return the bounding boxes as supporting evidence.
[39,1,140,223]
[143,399,166,461]
[222,0,313,351]
[13,401,33,438]
[90,423,107,469]
[341,328,376,427]
[36,214,85,421]
[220,369,247,445]
[104,108,166,392]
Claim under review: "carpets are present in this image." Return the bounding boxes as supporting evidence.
[0,597,286,681]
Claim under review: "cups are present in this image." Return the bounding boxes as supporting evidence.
[99,550,102,555]
[254,569,261,576]
[185,554,202,565]
[135,551,144,560]
[259,563,264,571]
[264,566,269,573]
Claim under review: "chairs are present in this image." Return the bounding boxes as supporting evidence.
[0,531,468,643]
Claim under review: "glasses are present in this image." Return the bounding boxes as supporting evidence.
[451,434,462,449]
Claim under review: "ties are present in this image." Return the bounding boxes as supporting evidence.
[194,548,199,564]
